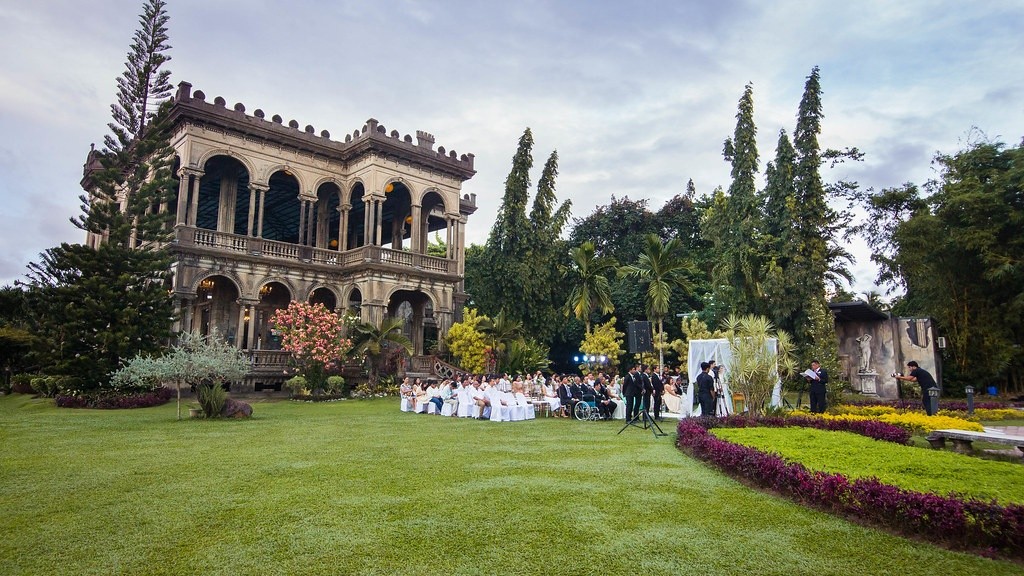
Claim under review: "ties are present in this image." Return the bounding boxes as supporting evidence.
[566,386,570,396]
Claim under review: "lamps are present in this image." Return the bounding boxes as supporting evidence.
[964,385,974,413]
[927,386,941,415]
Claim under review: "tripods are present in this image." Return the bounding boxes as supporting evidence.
[618,354,664,439]
[713,373,730,419]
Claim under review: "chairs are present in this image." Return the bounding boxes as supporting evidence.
[425,394,440,415]
[506,392,525,421]
[412,391,428,414]
[400,384,414,412]
[441,395,457,416]
[490,391,510,422]
[457,392,472,418]
[468,392,491,419]
[515,393,535,420]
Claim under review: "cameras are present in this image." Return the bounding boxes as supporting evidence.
[891,372,904,377]
[713,370,720,373]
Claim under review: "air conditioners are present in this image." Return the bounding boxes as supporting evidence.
[936,336,946,348]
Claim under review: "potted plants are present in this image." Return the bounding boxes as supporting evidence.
[185,404,202,418]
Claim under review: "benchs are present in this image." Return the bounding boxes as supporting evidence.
[531,399,580,420]
[924,429,1024,454]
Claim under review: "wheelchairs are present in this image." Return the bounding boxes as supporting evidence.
[575,392,605,423]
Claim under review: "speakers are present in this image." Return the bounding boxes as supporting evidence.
[629,321,654,354]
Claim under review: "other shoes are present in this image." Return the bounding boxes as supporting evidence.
[637,419,641,421]
[561,414,568,418]
[655,416,662,420]
[626,420,631,424]
[552,413,558,417]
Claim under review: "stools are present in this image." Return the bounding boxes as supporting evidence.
[732,394,745,413]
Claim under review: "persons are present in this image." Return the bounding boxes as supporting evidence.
[399,360,724,425]
[805,361,827,413]
[895,361,937,417]
[856,334,872,371]
